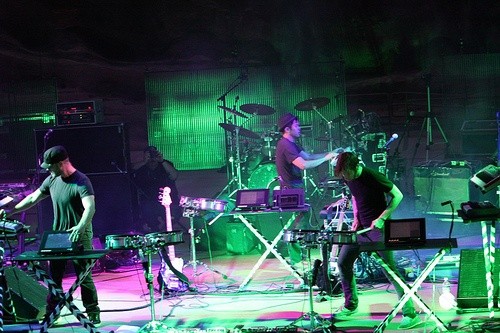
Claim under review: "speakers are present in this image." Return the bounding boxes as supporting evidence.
[33,123,140,240]
[413,164,475,216]
[456,248,500,308]
[0,266,48,323]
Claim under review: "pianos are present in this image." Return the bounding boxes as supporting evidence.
[358,238,458,333]
[13,251,115,333]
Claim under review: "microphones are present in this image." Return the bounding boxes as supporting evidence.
[245,62,249,81]
[384,134,398,147]
[46,129,53,136]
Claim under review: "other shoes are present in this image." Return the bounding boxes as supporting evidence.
[398,314,426,329]
[87,307,102,327]
[332,303,359,320]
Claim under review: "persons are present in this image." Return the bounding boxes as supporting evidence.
[330,153,422,329]
[0,145,102,325]
[132,145,184,233]
[275,112,342,270]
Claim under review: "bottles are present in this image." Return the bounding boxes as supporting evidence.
[440,278,452,309]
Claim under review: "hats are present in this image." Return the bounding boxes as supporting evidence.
[40,146,68,168]
[278,113,297,132]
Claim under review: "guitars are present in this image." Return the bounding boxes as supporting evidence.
[157,186,184,291]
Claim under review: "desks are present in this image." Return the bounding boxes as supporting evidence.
[12,249,110,333]
[227,205,308,289]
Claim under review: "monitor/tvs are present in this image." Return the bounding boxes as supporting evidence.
[38,231,74,252]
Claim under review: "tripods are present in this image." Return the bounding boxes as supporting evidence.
[288,244,336,333]
[302,170,321,200]
[216,75,259,202]
[181,208,236,285]
[411,78,449,166]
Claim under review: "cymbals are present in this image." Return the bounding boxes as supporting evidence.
[240,103,275,115]
[294,97,330,111]
[219,106,248,119]
[218,123,260,139]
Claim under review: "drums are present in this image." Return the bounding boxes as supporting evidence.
[179,196,195,209]
[282,228,321,246]
[322,231,358,245]
[246,154,264,170]
[267,177,280,203]
[247,161,278,189]
[262,141,276,156]
[145,230,185,247]
[196,198,229,212]
[105,234,143,250]
